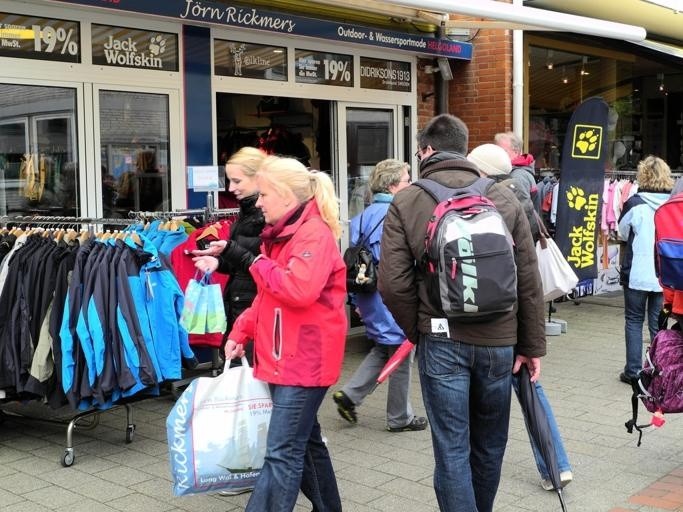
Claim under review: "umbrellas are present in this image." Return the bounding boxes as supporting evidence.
[518,364,570,512]
[368,339,414,395]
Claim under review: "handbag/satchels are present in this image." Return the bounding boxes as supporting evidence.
[164,366,274,498]
[177,276,227,335]
[534,237,580,303]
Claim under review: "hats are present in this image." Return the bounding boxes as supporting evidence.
[466,143,513,176]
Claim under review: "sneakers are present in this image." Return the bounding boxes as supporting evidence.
[620,373,634,384]
[540,469,572,492]
[333,390,357,425]
[386,414,428,431]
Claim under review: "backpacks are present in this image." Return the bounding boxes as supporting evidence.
[342,210,385,294]
[624,303,683,446]
[653,193,683,291]
[411,175,517,323]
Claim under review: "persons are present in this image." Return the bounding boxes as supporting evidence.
[494,132,542,247]
[330,157,428,431]
[192,145,270,497]
[22,149,164,213]
[192,155,348,512]
[378,113,547,512]
[617,154,675,385]
[651,174,683,333]
[467,144,573,491]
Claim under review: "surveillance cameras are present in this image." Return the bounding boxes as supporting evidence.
[438,57,454,83]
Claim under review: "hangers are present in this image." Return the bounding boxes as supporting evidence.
[0,208,241,235]
[539,170,560,178]
[672,172,679,179]
[604,170,637,181]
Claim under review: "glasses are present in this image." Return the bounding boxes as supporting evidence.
[395,179,411,184]
[415,145,435,162]
